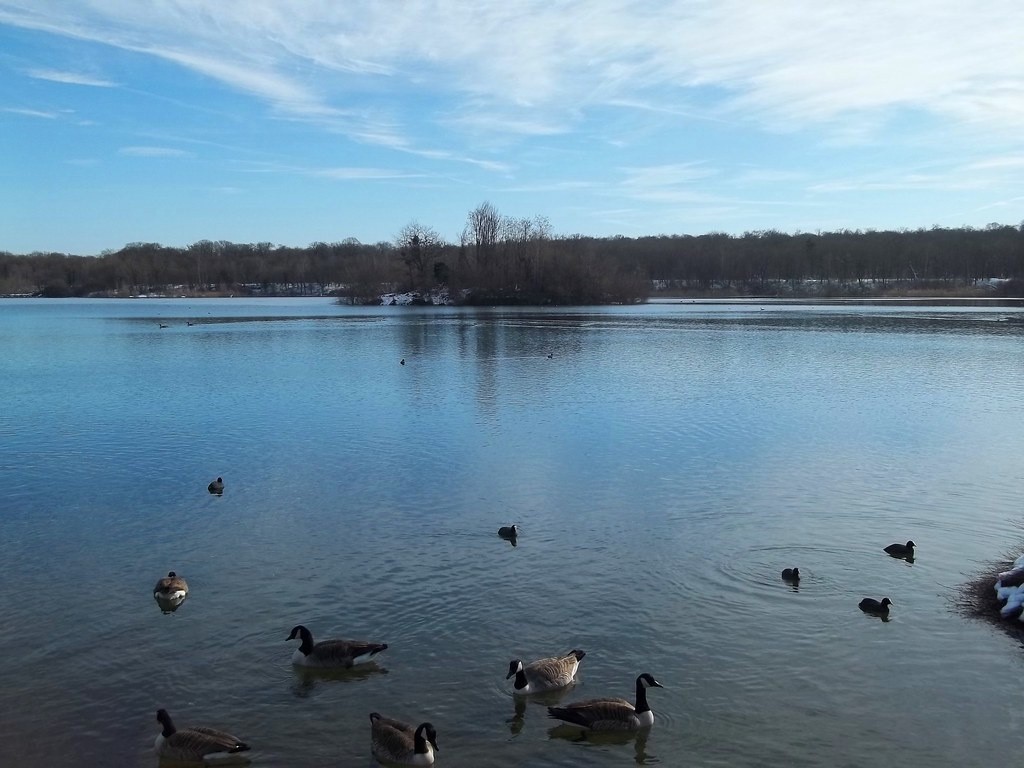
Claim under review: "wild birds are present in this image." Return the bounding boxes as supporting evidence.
[881,539,919,557]
[546,350,556,360]
[186,321,195,327]
[780,566,802,581]
[367,708,443,767]
[281,623,395,675]
[158,323,170,329]
[153,705,254,763]
[503,646,676,735]
[207,476,226,492]
[496,523,520,539]
[400,357,409,366]
[857,595,896,614]
[759,306,766,312]
[150,570,192,608]
